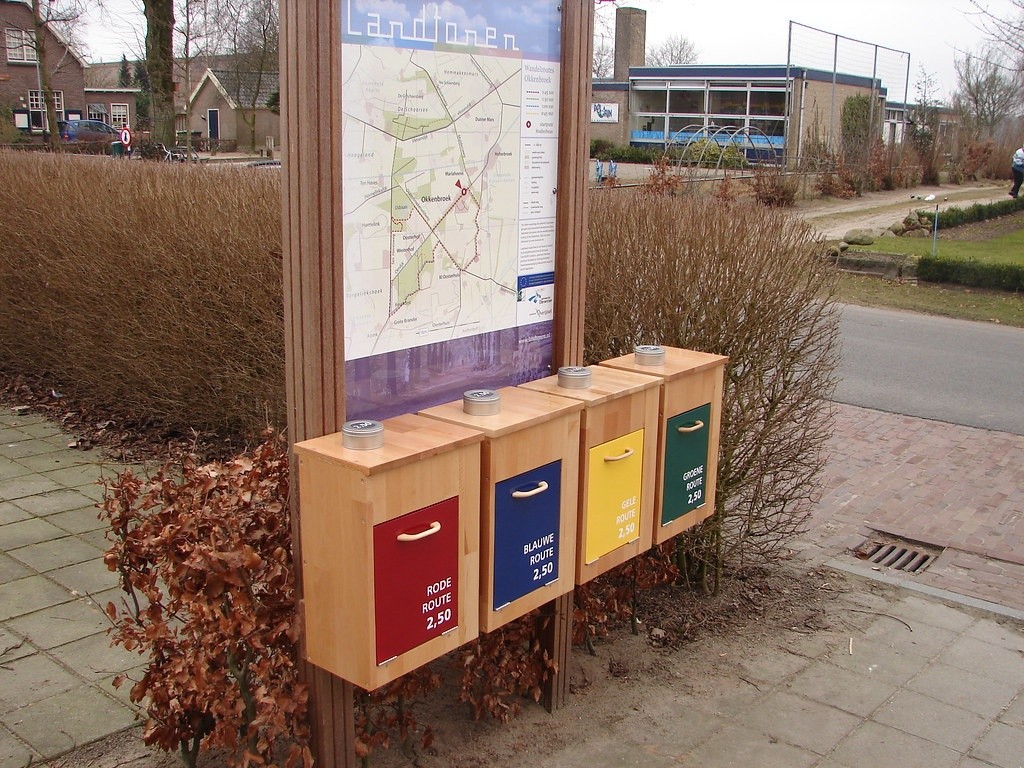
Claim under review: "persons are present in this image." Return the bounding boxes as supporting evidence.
[94,122,106,133]
[1008,145,1024,199]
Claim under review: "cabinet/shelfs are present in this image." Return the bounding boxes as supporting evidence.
[420,385,583,633]
[597,345,729,547]
[516,365,664,586]
[293,413,481,691]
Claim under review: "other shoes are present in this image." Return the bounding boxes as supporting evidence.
[1008,190,1018,198]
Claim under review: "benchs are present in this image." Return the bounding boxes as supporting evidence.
[173,146,209,164]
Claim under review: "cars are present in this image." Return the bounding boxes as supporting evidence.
[57,120,120,141]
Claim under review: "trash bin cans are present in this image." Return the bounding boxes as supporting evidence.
[598,344,730,546]
[517,364,665,585]
[420,385,584,633]
[292,413,485,691]
[111,141,124,158]
